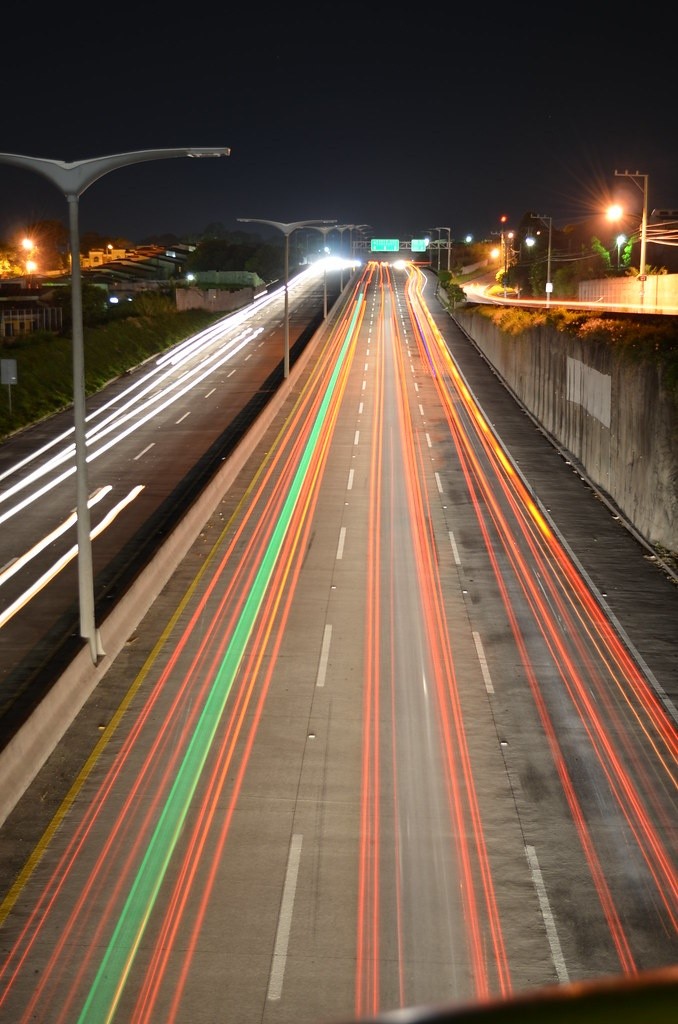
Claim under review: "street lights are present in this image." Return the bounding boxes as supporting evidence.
[525,236,553,311]
[410,226,451,275]
[605,204,648,311]
[0,146,232,667]
[237,218,338,381]
[295,224,372,320]
[499,215,514,303]
